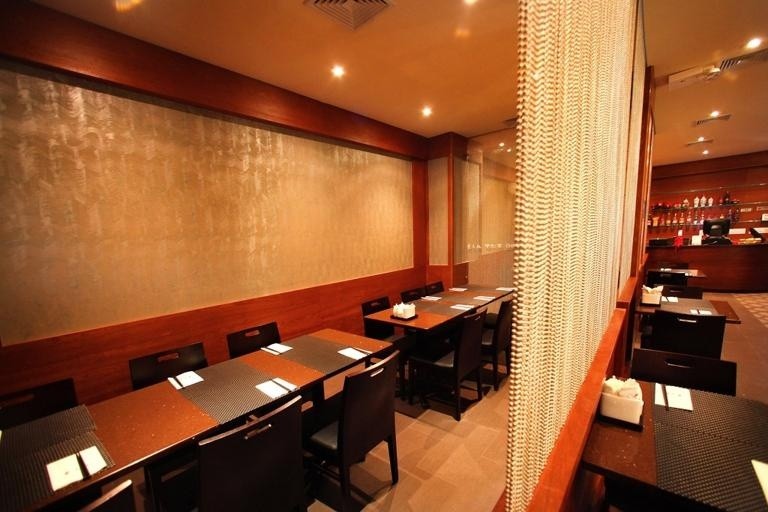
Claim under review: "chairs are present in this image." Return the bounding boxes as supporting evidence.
[629,259,737,396]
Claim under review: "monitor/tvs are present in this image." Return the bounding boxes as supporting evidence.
[703,218,730,237]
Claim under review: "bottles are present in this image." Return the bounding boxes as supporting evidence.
[392,302,416,320]
[646,192,740,227]
[603,375,644,402]
